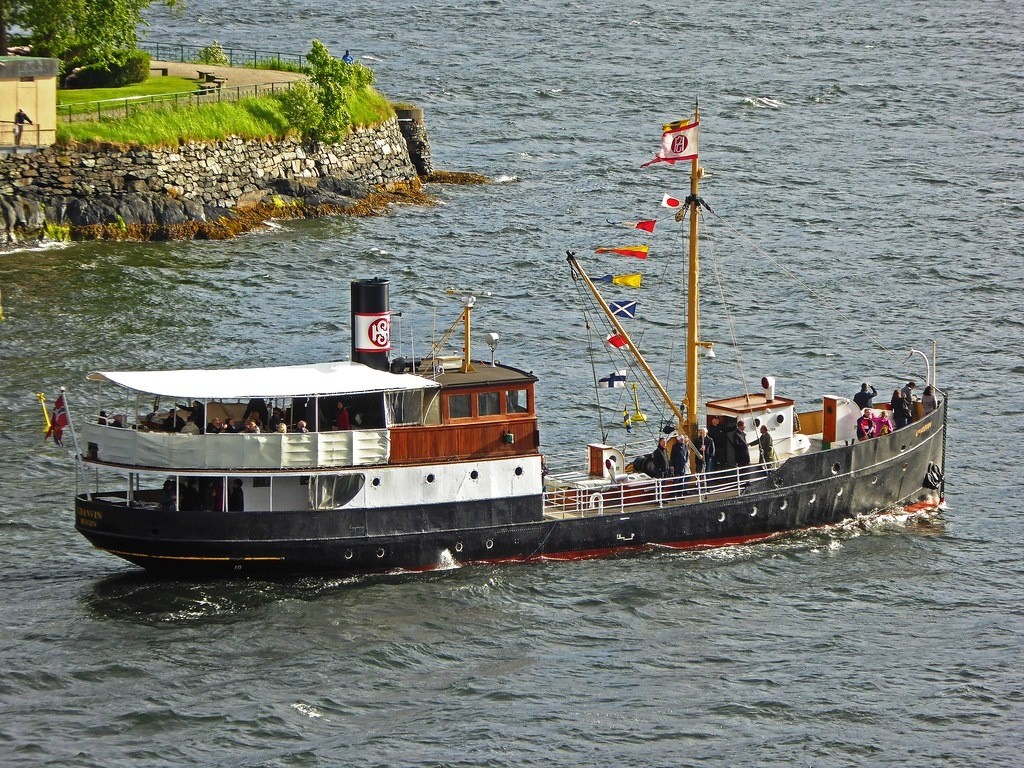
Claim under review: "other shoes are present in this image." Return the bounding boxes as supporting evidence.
[668,495,686,501]
[662,501,670,504]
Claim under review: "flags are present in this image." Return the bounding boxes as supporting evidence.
[609,301,636,318]
[597,370,626,389]
[607,330,630,350]
[641,119,699,168]
[660,193,684,209]
[46,395,69,448]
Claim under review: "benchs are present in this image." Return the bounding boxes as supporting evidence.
[197,69,226,95]
[149,67,168,76]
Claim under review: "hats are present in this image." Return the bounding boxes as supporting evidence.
[169,409,175,413]
[712,415,720,419]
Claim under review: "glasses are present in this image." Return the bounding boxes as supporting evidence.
[216,423,219,425]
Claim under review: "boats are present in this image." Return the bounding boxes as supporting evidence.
[75,89,948,595]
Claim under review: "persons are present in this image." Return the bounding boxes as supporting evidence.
[13,108,33,145]
[733,421,779,480]
[653,428,715,505]
[306,397,325,427]
[708,415,736,477]
[206,398,309,434]
[161,479,244,511]
[854,383,878,410]
[164,401,205,434]
[98,411,123,428]
[857,408,893,442]
[891,382,938,428]
[793,407,801,433]
[343,49,354,65]
[336,401,350,431]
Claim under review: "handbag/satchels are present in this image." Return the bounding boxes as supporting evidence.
[902,397,912,418]
